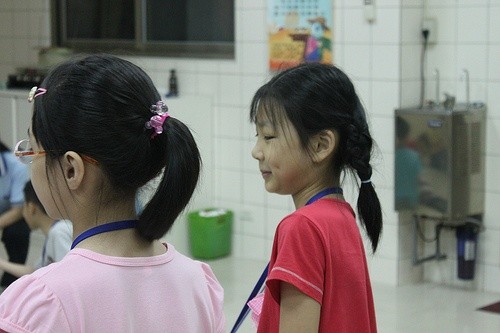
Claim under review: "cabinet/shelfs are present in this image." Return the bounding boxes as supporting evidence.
[0,96,213,251]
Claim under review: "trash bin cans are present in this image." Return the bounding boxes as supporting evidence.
[188,208,232,258]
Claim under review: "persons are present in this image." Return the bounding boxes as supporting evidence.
[231,63,383,333]
[0,53,224,333]
[0,180,74,278]
[0,140,31,288]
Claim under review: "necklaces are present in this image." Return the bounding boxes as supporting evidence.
[231,186,343,333]
[67,218,142,251]
[41,219,62,267]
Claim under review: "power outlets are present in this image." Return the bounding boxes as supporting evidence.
[420,19,436,44]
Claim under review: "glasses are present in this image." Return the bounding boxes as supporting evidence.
[14,139,100,167]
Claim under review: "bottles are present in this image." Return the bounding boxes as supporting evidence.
[170,70,178,96]
